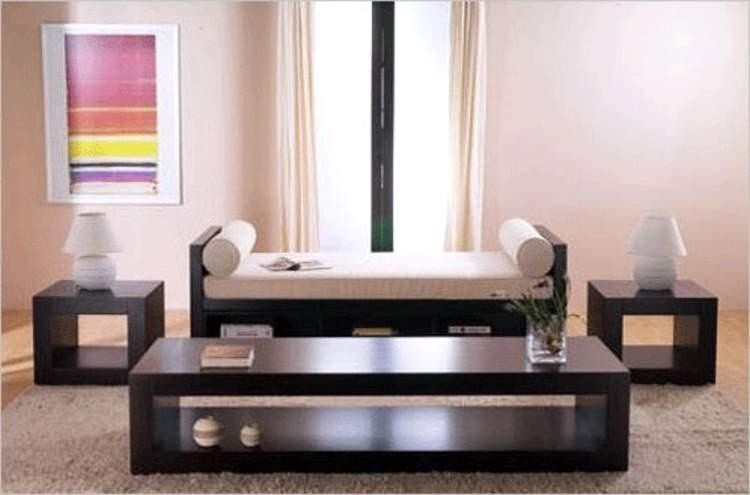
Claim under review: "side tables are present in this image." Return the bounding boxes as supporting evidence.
[587,280,719,385]
[32,282,164,385]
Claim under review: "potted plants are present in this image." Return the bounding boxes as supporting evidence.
[504,280,586,363]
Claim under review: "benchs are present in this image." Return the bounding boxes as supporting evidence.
[189,224,568,336]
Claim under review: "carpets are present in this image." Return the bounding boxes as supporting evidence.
[0,319,749,493]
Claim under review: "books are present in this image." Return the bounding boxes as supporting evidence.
[200,343,256,368]
[258,257,328,272]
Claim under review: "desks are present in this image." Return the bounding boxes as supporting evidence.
[129,335,629,473]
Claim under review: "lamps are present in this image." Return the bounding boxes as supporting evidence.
[627,213,686,290]
[60,211,126,290]
